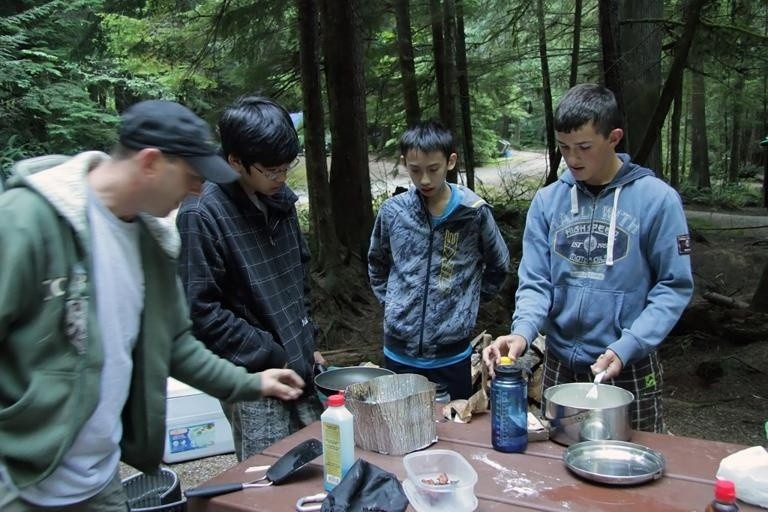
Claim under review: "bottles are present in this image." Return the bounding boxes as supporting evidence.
[704,481,741,512]
[490,357,533,453]
[435,385,450,424]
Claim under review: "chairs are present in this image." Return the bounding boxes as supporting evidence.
[125,497,188,510]
[114,466,181,512]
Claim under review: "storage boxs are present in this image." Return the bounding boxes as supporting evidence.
[161,375,236,466]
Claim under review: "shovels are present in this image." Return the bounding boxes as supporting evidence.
[184,438,323,499]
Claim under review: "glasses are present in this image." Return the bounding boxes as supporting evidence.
[251,157,299,180]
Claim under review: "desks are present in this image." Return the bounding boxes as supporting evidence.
[190,397,766,510]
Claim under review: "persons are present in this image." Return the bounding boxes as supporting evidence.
[482,83,696,435]
[176,96,327,458]
[366,120,510,405]
[2,101,305,510]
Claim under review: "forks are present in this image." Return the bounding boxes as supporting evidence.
[584,369,608,406]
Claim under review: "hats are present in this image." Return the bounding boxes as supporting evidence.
[120,100,241,184]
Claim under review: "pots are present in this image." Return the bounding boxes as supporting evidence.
[313,364,395,397]
